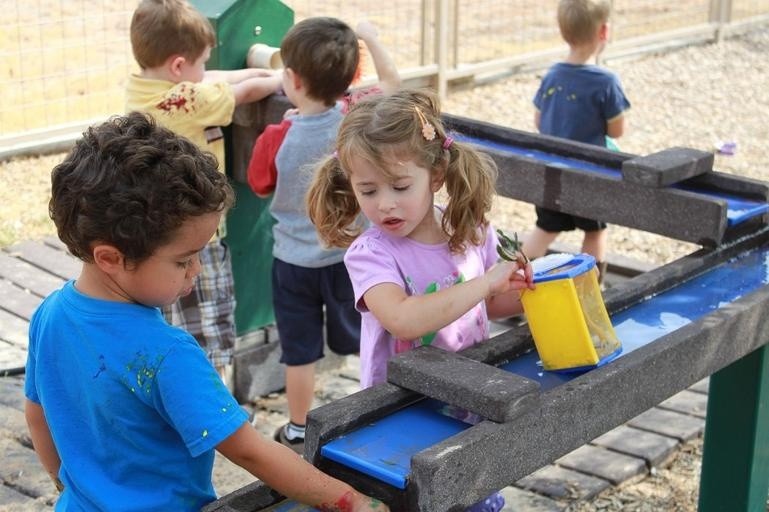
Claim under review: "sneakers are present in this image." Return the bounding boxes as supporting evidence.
[275,424,304,454]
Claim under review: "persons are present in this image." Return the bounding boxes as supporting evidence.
[246,15,406,454]
[514,0,634,263]
[18,111,392,512]
[121,0,285,388]
[299,87,601,509]
